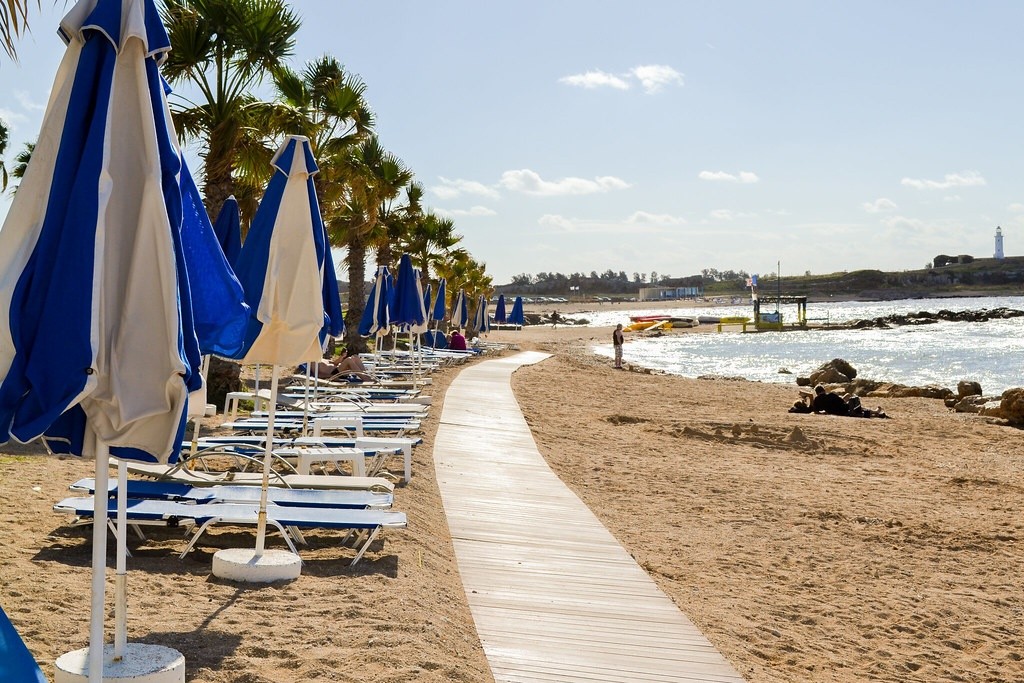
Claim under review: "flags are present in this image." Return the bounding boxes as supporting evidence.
[575,286,580,290]
[746,277,757,286]
[570,286,574,290]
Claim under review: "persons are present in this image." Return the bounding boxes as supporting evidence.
[551,311,558,329]
[358,266,392,365]
[790,384,881,417]
[301,349,373,382]
[449,330,467,350]
[613,324,624,369]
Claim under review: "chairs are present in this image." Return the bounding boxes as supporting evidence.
[53,341,522,558]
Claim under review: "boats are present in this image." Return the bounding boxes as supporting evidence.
[623,314,751,332]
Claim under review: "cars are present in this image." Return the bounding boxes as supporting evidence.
[591,296,638,302]
[484,296,568,303]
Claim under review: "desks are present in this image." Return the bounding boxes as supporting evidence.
[359,352,380,361]
[224,390,269,424]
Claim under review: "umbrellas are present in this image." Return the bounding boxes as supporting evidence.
[415,284,431,346]
[493,294,506,330]
[0,0,249,683]
[507,296,524,332]
[432,278,446,350]
[188,195,241,469]
[390,254,426,390]
[237,135,342,555]
[451,290,468,333]
[473,294,490,346]
